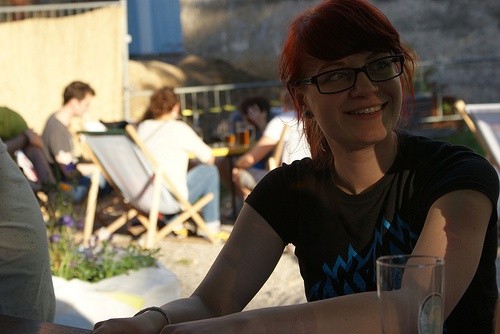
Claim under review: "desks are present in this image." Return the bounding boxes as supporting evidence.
[186,141,258,221]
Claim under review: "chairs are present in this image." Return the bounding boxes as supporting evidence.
[68,123,218,249]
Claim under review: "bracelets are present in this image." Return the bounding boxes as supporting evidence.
[135,304,172,326]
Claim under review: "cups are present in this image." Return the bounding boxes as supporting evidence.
[376,255,445,334]
[236,121,249,145]
[224,126,236,145]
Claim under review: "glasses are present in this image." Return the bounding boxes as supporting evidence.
[296,53,408,95]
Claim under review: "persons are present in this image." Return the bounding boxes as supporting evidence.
[43,81,111,203]
[91,0,500,334]
[0,139,56,323]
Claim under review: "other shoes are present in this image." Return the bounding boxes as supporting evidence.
[196,220,221,235]
